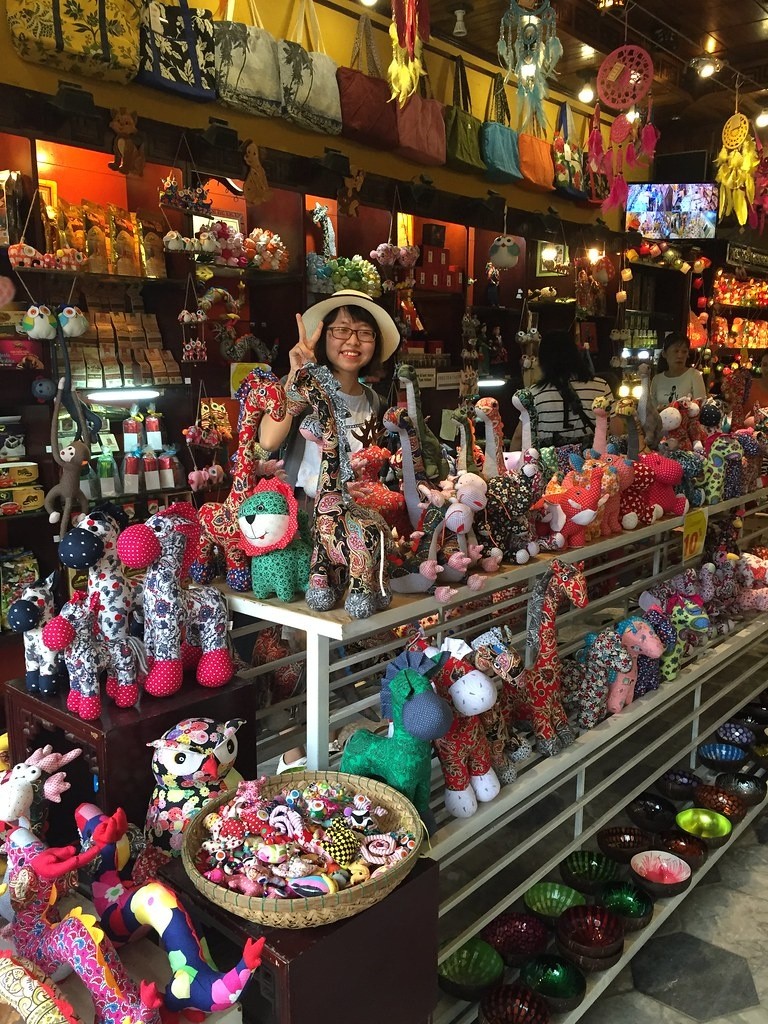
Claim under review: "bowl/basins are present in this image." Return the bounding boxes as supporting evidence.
[440,701,768,1024]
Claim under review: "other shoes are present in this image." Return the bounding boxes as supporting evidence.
[276,754,308,775]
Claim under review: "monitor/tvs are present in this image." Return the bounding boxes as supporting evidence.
[622,180,720,244]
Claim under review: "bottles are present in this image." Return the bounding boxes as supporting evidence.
[627,329,631,348]
[650,330,654,349]
[642,330,647,349]
[638,330,642,349]
[633,329,638,349]
[654,331,657,348]
[647,330,651,349]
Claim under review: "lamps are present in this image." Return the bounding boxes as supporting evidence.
[592,215,612,240]
[691,52,724,77]
[540,205,562,235]
[410,173,436,205]
[318,143,352,178]
[52,78,102,120]
[481,186,506,216]
[626,226,642,244]
[202,116,240,153]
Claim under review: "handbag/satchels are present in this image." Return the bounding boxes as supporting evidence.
[8,1,613,207]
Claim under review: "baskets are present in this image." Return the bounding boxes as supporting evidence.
[181,770,431,928]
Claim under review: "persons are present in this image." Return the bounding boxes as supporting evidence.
[259,290,400,775]
[650,331,706,406]
[510,328,624,451]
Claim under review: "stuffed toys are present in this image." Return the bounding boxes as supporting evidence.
[9,244,89,272]
[309,202,337,258]
[592,256,615,283]
[198,286,279,365]
[489,236,520,269]
[22,303,88,339]
[0,367,768,1024]
[370,243,420,268]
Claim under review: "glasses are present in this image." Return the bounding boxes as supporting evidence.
[326,327,377,343]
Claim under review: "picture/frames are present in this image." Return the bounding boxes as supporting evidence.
[37,179,57,208]
[192,208,246,244]
[536,241,571,277]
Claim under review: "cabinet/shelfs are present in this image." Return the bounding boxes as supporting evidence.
[1,484,768,1024]
[0,122,625,1024]
[703,241,768,363]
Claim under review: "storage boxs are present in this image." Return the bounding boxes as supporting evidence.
[413,224,465,294]
[403,341,445,354]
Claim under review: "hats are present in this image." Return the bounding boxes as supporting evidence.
[297,287,400,362]
